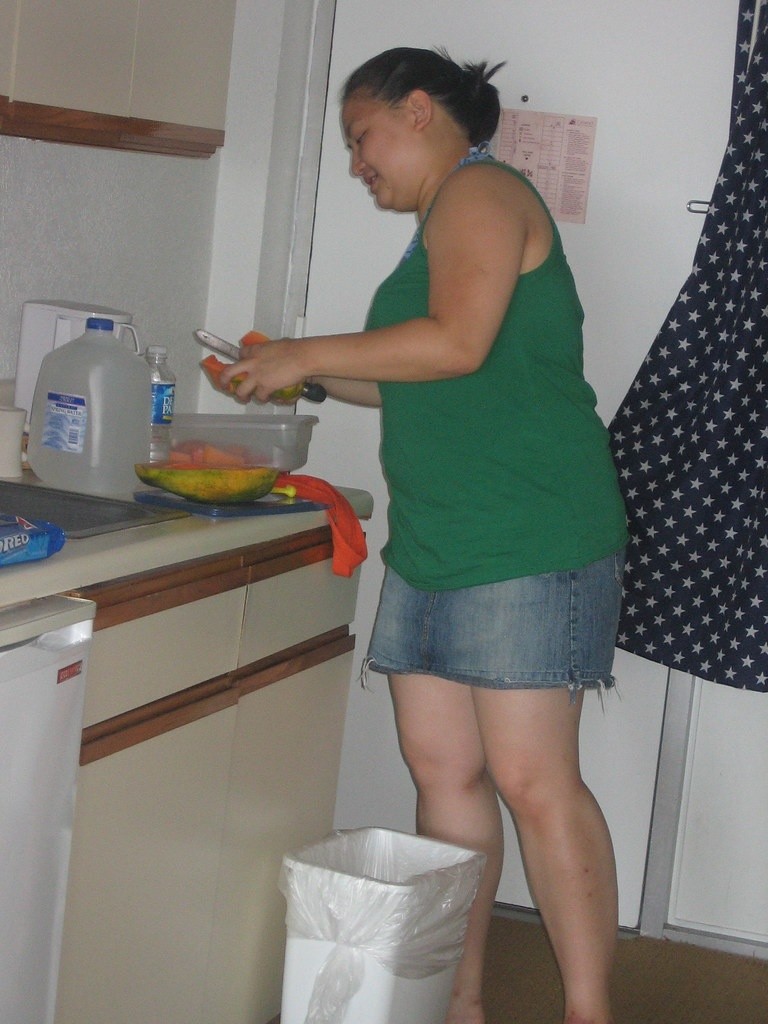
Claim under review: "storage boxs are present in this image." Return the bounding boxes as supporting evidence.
[170,413,322,471]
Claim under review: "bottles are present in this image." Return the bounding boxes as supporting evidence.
[146,345,175,462]
[27,319,152,496]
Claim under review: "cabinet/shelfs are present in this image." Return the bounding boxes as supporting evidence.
[0,485,376,1024]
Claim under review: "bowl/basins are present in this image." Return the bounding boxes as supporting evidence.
[170,414,318,473]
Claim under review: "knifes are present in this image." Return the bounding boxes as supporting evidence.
[196,330,327,402]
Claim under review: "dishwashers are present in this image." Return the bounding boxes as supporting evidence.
[0,596,96,1024]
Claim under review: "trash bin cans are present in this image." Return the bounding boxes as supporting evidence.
[280,824,487,1024]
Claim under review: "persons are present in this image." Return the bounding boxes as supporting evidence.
[221,45,627,1024]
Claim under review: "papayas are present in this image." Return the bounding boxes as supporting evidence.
[167,440,253,467]
[198,330,305,403]
[133,462,279,503]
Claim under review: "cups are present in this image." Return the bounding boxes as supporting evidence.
[0,406,27,477]
